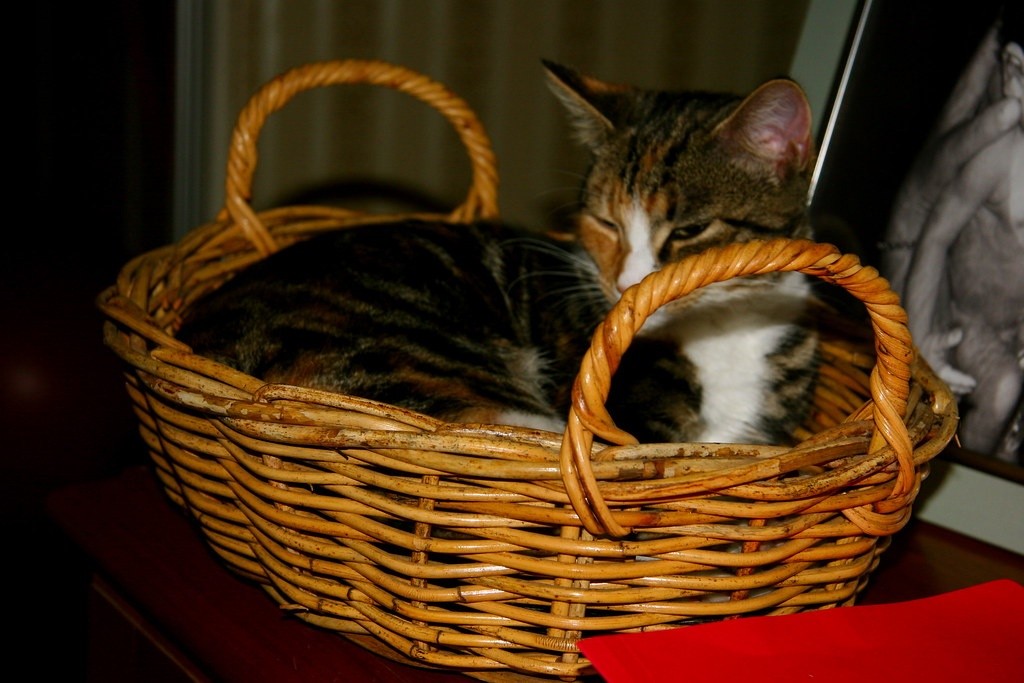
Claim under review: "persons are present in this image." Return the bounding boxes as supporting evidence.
[874,11,1023,473]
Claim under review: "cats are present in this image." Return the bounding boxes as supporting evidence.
[173,58,819,444]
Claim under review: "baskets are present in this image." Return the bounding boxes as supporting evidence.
[97,60,960,683]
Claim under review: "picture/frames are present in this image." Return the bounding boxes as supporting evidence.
[800,0,1024,485]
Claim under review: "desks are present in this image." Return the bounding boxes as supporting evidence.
[36,450,1023,682]
